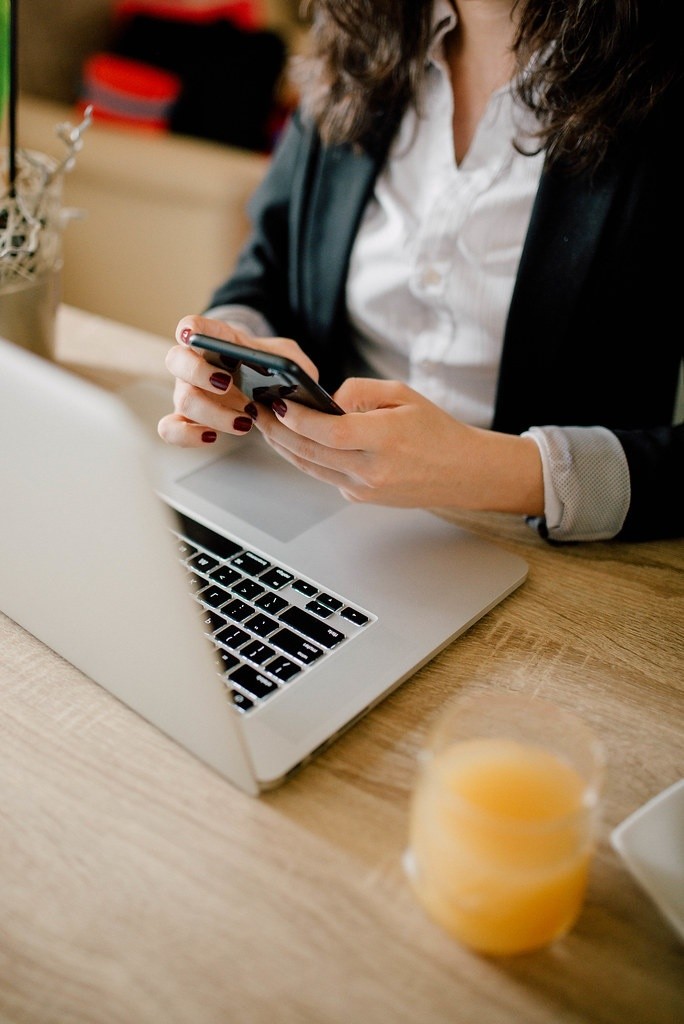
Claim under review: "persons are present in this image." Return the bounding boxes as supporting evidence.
[156,0,683,549]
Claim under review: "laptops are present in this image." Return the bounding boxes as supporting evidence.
[2,335,529,798]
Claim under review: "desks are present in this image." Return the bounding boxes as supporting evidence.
[0,304,684,1024]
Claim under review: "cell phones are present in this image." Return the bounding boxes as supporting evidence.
[186,335,351,421]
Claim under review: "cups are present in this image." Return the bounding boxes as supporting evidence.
[403,696,604,955]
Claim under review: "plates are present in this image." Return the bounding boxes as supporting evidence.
[609,777,684,938]
